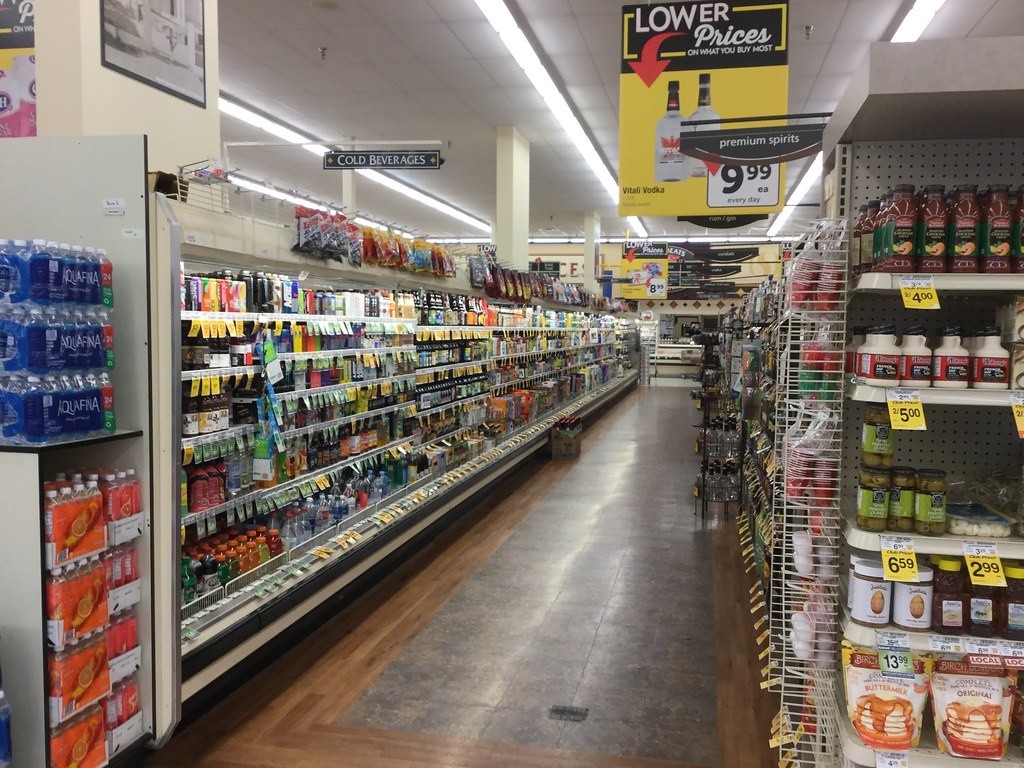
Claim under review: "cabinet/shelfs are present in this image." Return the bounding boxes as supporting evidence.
[849,267,1024,768]
[1,429,155,767]
[181,309,641,725]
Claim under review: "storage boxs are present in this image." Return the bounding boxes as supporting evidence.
[43,514,142,767]
[551,428,580,459]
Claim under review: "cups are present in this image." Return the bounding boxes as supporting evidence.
[781,436,838,666]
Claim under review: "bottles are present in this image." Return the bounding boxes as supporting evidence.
[845,327,1010,390]
[179,324,417,608]
[0,691,12,765]
[694,416,742,457]
[180,261,414,319]
[489,329,586,383]
[786,257,843,400]
[417,340,489,474]
[488,304,589,327]
[850,182,1024,279]
[414,287,486,325]
[693,459,742,501]
[37,466,138,739]
[744,272,780,325]
[0,235,115,449]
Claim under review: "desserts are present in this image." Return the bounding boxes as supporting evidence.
[942,697,1004,759]
[854,689,915,749]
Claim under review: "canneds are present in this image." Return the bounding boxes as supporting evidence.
[847,552,935,633]
[855,402,946,538]
